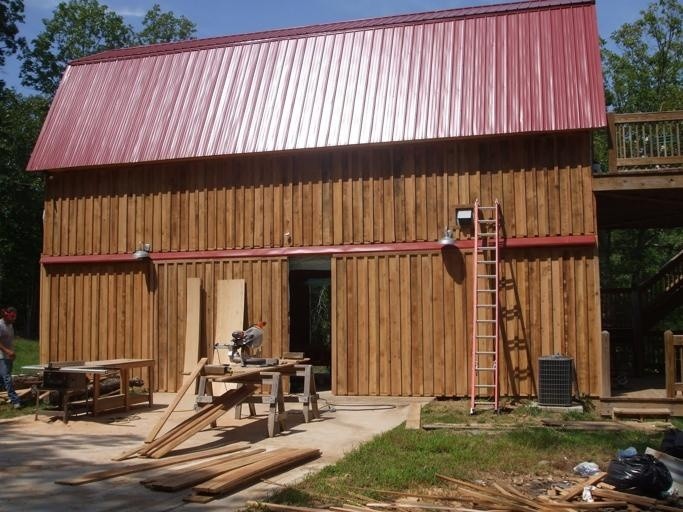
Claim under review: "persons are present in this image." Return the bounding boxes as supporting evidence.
[0,306,24,409]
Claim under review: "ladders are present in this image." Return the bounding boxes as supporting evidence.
[470,197,498,415]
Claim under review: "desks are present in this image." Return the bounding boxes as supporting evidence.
[79,358,156,419]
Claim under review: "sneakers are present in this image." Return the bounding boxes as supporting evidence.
[13,401,21,409]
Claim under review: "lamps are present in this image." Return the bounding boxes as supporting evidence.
[441,226,458,248]
[134,241,150,261]
